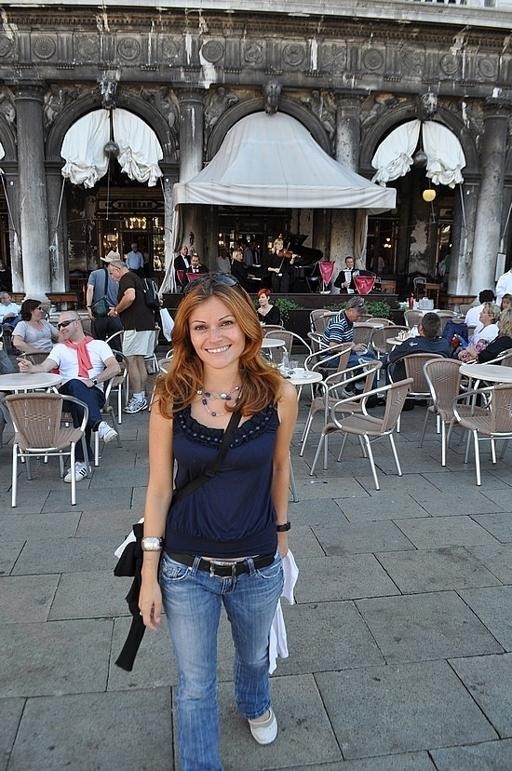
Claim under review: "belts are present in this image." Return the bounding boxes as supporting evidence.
[166,550,274,578]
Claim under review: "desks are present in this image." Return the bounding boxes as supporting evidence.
[1,372,64,479]
[446,365,512,469]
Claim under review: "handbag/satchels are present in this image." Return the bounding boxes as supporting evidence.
[116,527,145,617]
[91,298,112,319]
[443,318,468,344]
[459,339,489,362]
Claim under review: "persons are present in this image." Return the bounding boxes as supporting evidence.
[254,288,280,325]
[383,267,512,412]
[319,295,379,407]
[174,238,360,295]
[0,243,158,483]
[135,272,298,771]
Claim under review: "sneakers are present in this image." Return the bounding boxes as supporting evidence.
[64,462,91,483]
[123,394,148,414]
[98,421,118,444]
[247,709,278,744]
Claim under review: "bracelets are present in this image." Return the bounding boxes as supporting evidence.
[141,536,162,551]
[277,522,291,532]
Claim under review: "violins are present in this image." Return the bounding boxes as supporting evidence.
[278,249,301,260]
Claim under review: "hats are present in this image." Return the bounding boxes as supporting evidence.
[100,250,121,264]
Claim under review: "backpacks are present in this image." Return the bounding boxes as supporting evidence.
[140,278,166,315]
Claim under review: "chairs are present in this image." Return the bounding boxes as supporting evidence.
[309,378,415,491]
[299,361,382,457]
[1,277,512,404]
[443,382,511,485]
[417,358,490,467]
[4,394,88,509]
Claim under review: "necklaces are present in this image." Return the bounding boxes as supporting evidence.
[195,386,241,418]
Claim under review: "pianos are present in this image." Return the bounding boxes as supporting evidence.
[249,264,313,293]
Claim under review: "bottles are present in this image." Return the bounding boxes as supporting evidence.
[397,330,406,340]
[410,325,418,337]
[406,293,416,311]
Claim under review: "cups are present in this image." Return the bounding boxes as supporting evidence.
[282,351,298,371]
[270,362,278,369]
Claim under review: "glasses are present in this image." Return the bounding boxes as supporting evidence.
[182,272,246,296]
[57,319,76,331]
